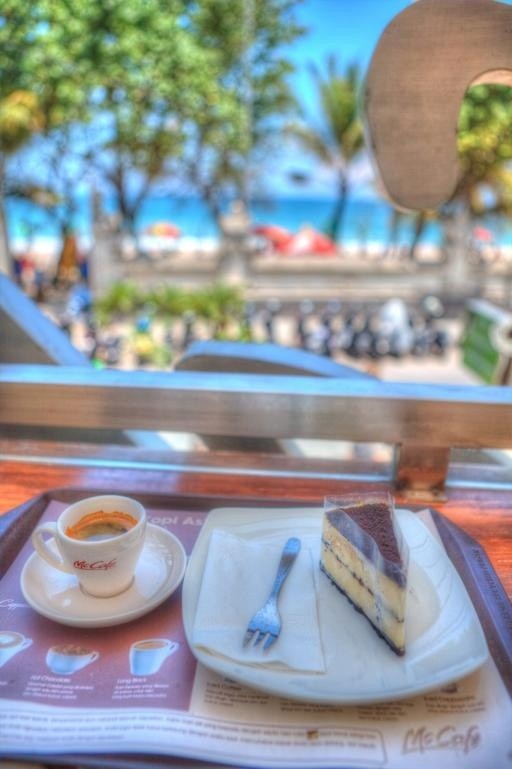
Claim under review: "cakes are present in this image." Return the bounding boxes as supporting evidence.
[319,501,412,655]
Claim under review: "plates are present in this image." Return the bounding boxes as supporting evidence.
[19,522,189,629]
[180,503,490,709]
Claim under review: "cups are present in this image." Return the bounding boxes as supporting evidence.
[46,643,102,677]
[128,638,179,677]
[28,493,149,598]
[0,630,32,669]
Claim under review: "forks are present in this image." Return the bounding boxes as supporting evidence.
[239,536,302,653]
